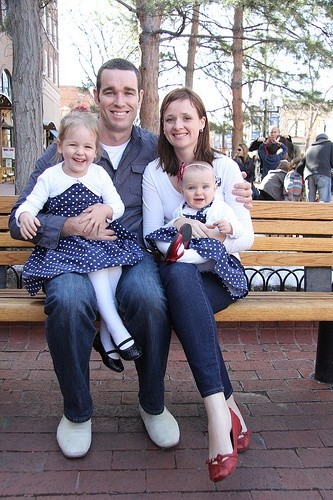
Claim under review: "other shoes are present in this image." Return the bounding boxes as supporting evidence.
[140,403,180,449]
[56,414,93,458]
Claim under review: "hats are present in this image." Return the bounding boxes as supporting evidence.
[315,134,328,141]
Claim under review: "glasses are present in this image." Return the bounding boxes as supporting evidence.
[237,149,243,151]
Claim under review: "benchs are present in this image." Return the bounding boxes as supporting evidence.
[0,194,333,383]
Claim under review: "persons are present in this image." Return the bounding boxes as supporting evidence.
[296,134,333,203]
[145,161,249,300]
[15,110,145,373]
[232,124,305,202]
[142,87,255,482]
[8,58,253,458]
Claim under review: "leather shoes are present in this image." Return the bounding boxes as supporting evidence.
[179,223,192,250]
[164,233,185,263]
[110,332,143,362]
[206,408,242,482]
[234,400,251,453]
[93,331,124,373]
